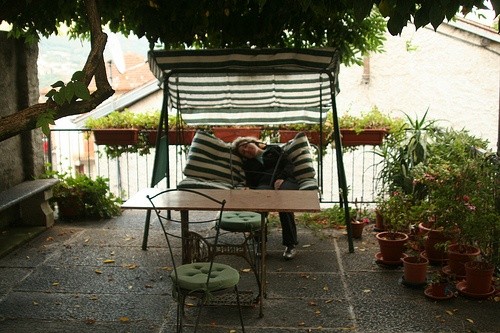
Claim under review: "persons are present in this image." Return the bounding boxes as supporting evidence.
[232,137,299,259]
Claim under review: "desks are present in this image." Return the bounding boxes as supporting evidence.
[120,188,320,318]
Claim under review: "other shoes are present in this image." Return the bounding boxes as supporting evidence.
[283,244,297,260]
[255,242,262,258]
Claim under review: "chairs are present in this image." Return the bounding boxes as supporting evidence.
[147,189,246,333]
[205,141,285,267]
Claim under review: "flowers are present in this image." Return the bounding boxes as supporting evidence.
[375,149,500,283]
[350,198,372,224]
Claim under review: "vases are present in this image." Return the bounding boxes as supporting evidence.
[353,221,365,239]
[464,260,493,293]
[418,222,456,258]
[376,231,408,260]
[402,255,430,282]
[376,212,383,228]
[431,284,449,298]
[445,243,481,275]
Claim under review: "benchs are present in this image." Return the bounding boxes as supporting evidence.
[0,178,60,228]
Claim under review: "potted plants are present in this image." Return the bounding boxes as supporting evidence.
[30,161,127,222]
[84,111,406,157]
